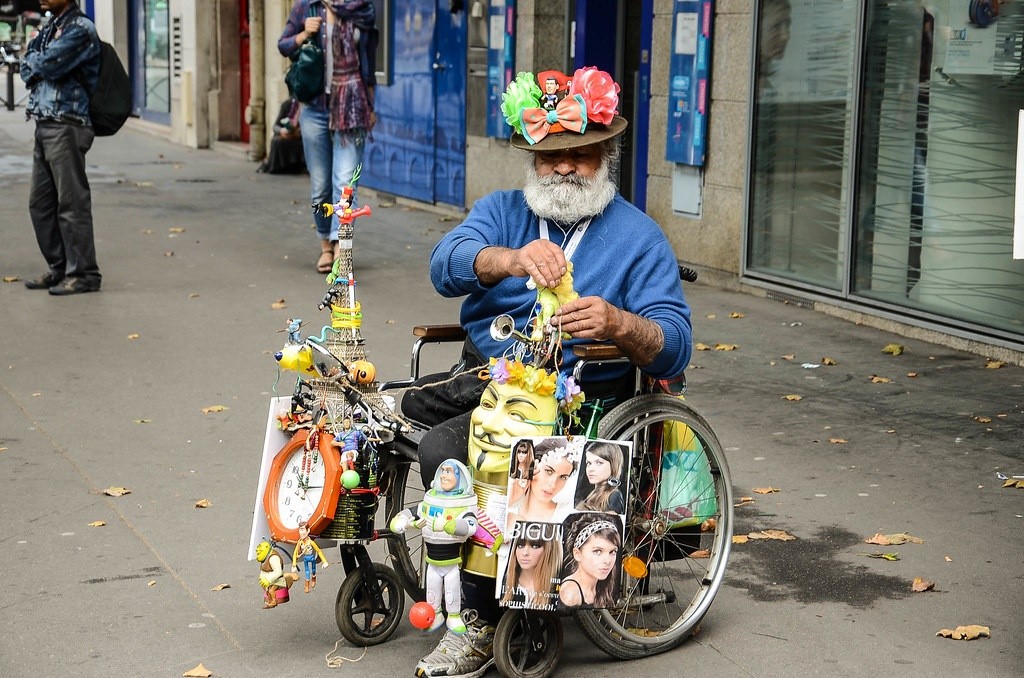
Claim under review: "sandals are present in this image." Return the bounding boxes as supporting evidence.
[315,249,334,273]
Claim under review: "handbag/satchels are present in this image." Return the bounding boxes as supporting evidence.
[283,2,324,103]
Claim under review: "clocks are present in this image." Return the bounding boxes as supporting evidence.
[262,432,343,546]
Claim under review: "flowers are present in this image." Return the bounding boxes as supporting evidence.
[500,66,620,143]
[486,358,589,430]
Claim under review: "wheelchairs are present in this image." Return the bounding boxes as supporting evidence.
[334,264,733,678]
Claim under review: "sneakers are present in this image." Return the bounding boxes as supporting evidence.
[48,274,99,294]
[22,263,64,289]
[413,606,522,678]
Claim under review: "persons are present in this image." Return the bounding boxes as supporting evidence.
[499,439,625,610]
[278,0,380,271]
[292,522,328,592]
[285,317,303,344]
[400,68,693,678]
[391,460,476,633]
[330,418,381,473]
[466,360,585,515]
[759,3,791,88]
[295,409,328,500]
[20,0,102,293]
[858,0,935,297]
[541,78,560,108]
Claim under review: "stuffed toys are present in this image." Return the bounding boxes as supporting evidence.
[525,263,578,341]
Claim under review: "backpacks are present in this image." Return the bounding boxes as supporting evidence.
[63,13,133,137]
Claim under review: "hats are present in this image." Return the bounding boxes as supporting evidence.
[499,66,628,151]
